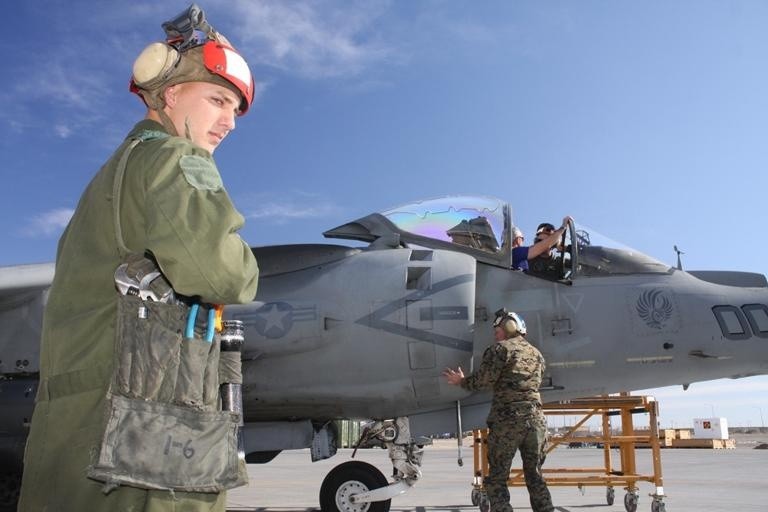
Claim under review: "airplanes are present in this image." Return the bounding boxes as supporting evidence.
[0,195,767,512]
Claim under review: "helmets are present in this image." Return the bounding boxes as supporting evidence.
[491,307,527,336]
[127,1,256,117]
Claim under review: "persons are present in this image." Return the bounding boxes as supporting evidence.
[440,308,555,511]
[509,215,575,272]
[16,6,262,512]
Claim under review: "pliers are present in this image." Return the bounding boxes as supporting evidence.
[185,304,215,341]
